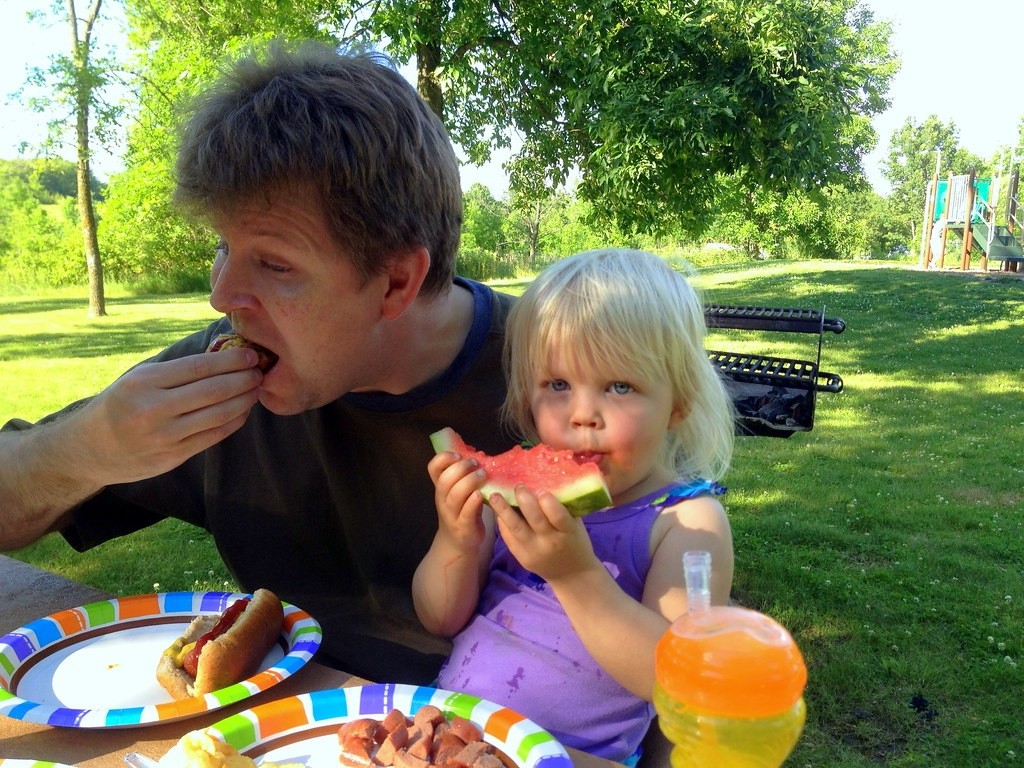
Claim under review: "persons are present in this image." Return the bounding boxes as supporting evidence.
[0,47,523,687]
[415,247,733,768]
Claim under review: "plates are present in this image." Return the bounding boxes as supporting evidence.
[210,684,573,768]
[0,592,323,729]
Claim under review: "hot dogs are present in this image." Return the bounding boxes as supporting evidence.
[156,589,283,700]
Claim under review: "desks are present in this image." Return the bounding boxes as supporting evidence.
[0,551,628,768]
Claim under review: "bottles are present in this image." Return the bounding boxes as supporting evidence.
[653,549,809,768]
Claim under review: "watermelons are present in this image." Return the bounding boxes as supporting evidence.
[430,428,612,518]
